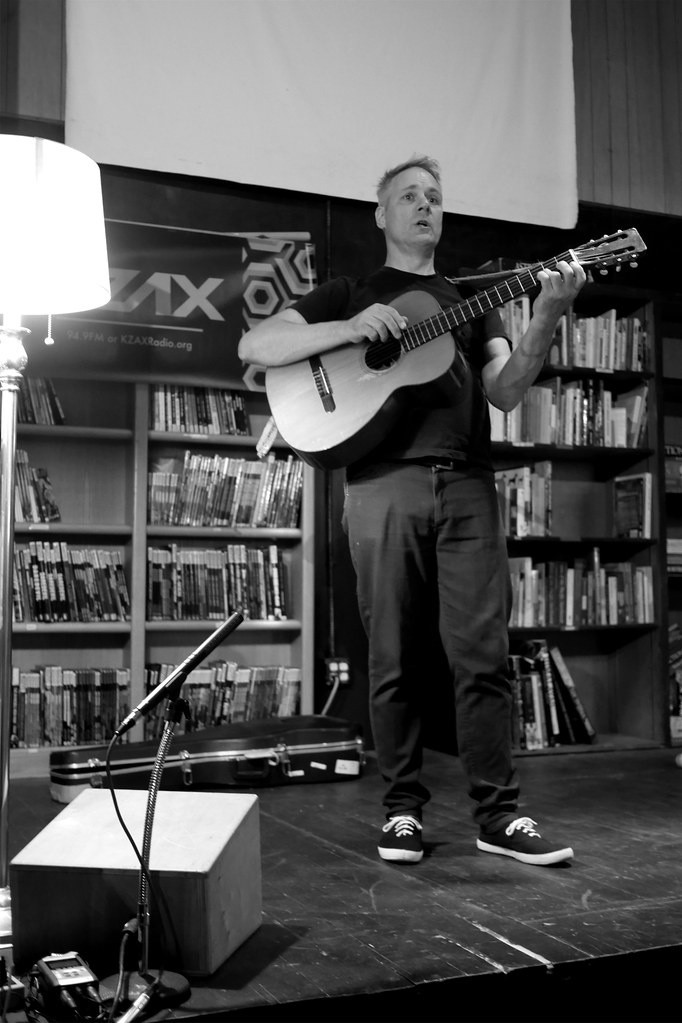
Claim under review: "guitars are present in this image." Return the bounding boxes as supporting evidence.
[259,227,647,474]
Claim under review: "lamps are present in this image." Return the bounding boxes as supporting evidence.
[0,134,112,942]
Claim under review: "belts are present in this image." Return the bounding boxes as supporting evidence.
[386,456,464,472]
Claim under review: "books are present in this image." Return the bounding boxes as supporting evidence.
[498,296,645,371]
[146,660,302,740]
[11,664,131,749]
[12,541,131,622]
[14,449,61,522]
[494,462,652,539]
[490,376,649,449]
[662,331,682,737]
[507,548,654,626]
[148,449,302,529]
[510,640,596,750]
[17,378,251,435]
[148,543,288,621]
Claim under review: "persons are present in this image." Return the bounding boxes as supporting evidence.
[236,156,576,869]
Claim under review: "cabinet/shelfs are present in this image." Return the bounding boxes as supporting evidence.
[487,295,681,755]
[1,362,315,779]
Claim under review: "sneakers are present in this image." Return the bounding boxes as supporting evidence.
[378,814,423,862]
[476,817,574,865]
